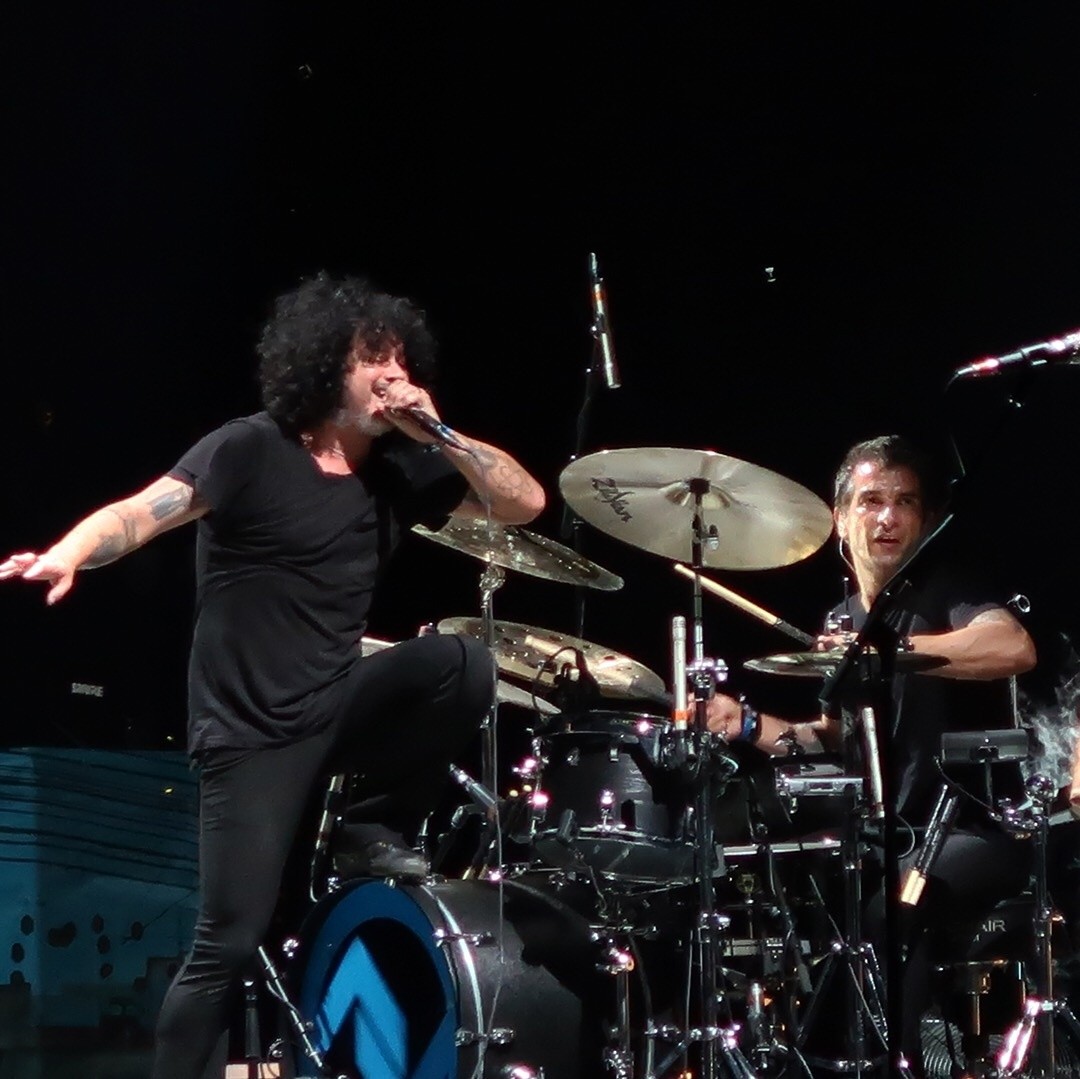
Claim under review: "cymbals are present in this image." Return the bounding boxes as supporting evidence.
[741,651,954,681]
[410,495,626,594]
[559,447,834,572]
[434,615,677,706]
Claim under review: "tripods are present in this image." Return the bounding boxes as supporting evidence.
[464,499,1080,1079]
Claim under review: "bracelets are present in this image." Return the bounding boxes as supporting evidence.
[734,705,761,744]
[422,424,452,453]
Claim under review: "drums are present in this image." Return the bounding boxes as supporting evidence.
[273,875,631,1079]
[701,838,856,964]
[347,634,563,727]
[518,706,709,886]
[745,755,885,837]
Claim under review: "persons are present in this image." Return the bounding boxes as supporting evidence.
[0,272,546,1079]
[688,435,1035,1002]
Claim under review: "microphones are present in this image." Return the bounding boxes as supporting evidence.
[309,773,343,867]
[246,982,261,1060]
[370,377,460,449]
[588,251,621,388]
[576,650,601,701]
[956,330,1080,378]
